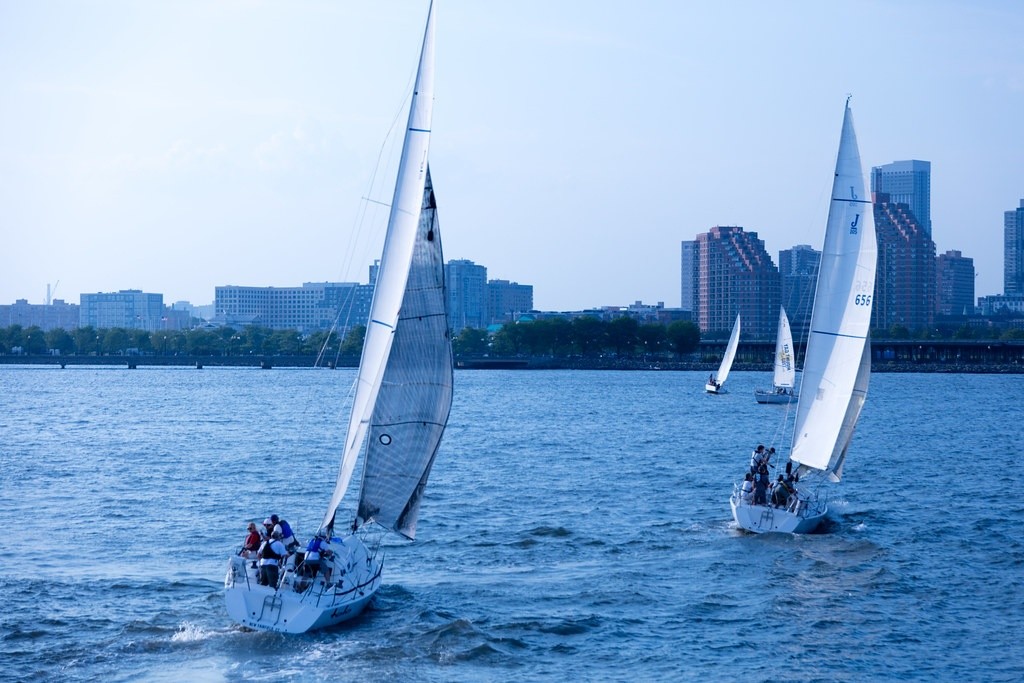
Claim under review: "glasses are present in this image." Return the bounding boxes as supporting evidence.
[247,527,253,530]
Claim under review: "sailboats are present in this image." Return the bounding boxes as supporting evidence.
[221,2,460,637]
[702,312,742,396]
[716,95,878,543]
[754,304,801,405]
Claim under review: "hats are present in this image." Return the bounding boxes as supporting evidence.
[263,519,272,524]
[271,531,279,537]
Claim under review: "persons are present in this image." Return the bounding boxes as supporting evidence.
[769,474,783,507]
[754,463,769,505]
[257,531,290,590]
[708,373,720,390]
[298,529,331,594]
[775,387,786,395]
[750,445,770,476]
[242,523,260,559]
[741,474,756,505]
[775,474,795,510]
[260,518,275,541]
[270,515,301,565]
